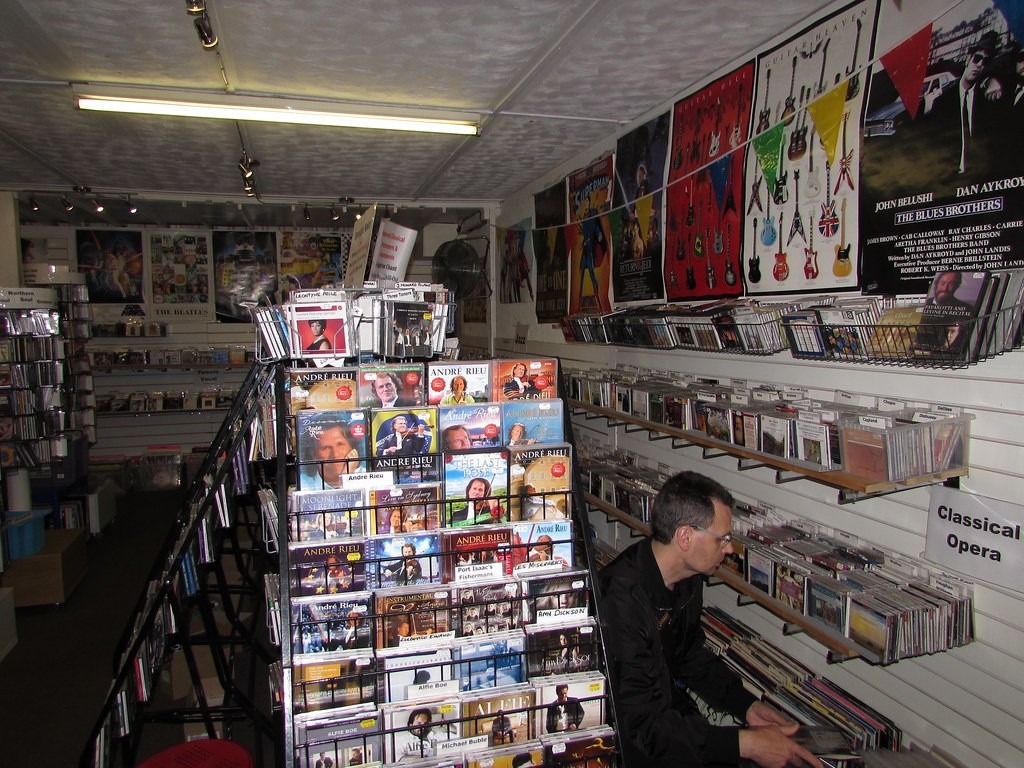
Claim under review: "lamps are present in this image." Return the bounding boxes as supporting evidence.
[128,202,138,214]
[194,18,218,48]
[185,0,205,11]
[356,207,362,220]
[28,196,39,210]
[385,210,390,219]
[61,198,73,211]
[238,162,255,197]
[303,208,311,219]
[72,84,482,138]
[330,209,339,220]
[91,199,104,212]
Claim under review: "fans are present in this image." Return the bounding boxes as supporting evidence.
[431,236,492,300]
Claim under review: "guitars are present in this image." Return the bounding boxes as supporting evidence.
[787,85,811,162]
[760,186,777,246]
[818,160,839,238]
[773,212,789,282]
[779,56,796,125]
[786,169,807,247]
[804,125,821,198]
[812,37,832,102]
[833,111,855,195]
[756,68,770,135]
[803,217,818,280]
[745,156,764,216]
[747,216,762,283]
[774,133,789,205]
[833,198,851,277]
[843,18,864,101]
[667,85,735,290]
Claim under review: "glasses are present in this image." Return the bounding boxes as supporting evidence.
[689,524,733,550]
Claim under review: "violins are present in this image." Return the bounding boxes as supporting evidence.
[479,495,508,507]
[520,371,549,383]
[515,438,543,445]
[401,423,434,436]
[406,560,420,575]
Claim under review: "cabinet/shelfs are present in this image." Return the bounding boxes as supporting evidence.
[0,283,1024,768]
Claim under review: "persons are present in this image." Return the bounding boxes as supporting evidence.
[546,684,584,734]
[316,749,362,768]
[21,237,34,264]
[293,590,591,650]
[302,414,536,490]
[413,670,429,684]
[151,234,209,304]
[401,708,438,756]
[492,710,514,745]
[777,563,803,609]
[90,250,136,298]
[622,164,654,299]
[144,455,165,465]
[393,319,432,357]
[918,269,973,353]
[321,535,553,592]
[500,245,534,303]
[462,667,506,691]
[292,479,570,542]
[361,363,540,408]
[553,632,578,673]
[590,470,825,768]
[576,195,607,315]
[305,319,332,350]
